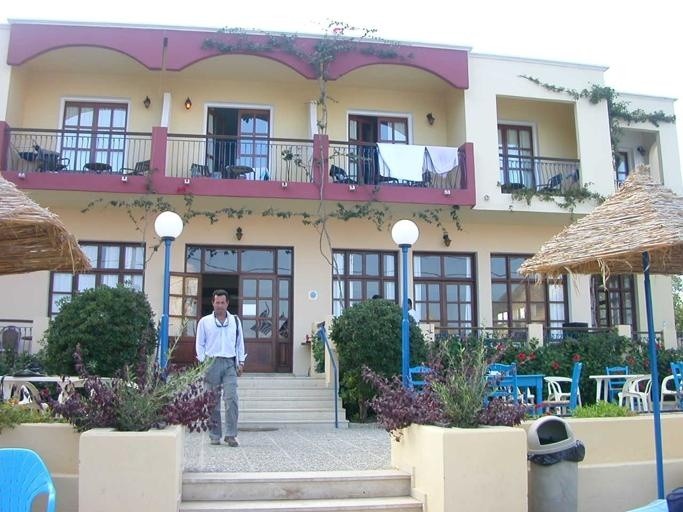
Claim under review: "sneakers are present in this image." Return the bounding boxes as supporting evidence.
[224,436,238,446]
[211,439,220,445]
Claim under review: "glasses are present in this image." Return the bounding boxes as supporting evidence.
[215,317,229,327]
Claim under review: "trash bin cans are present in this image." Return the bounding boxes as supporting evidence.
[526,415,578,512]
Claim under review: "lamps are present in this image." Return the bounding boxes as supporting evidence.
[426,112,436,125]
[184,96,193,112]
[143,95,152,109]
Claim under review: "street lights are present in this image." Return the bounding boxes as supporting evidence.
[391,218,421,387]
[154,210,184,370]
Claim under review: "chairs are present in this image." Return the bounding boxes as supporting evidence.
[0,325,20,367]
[190,164,213,179]
[119,160,150,176]
[0,448,57,512]
[0,376,139,419]
[38,149,70,172]
[480,361,682,418]
[407,363,436,394]
[329,165,357,184]
[533,173,562,193]
[405,170,432,187]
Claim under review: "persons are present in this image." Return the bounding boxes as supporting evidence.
[193,289,245,449]
[406,298,419,323]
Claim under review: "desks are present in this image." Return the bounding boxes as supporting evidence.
[82,163,112,174]
[500,183,525,194]
[380,176,400,185]
[225,165,256,180]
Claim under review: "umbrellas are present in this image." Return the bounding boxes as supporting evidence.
[518,163,683,498]
